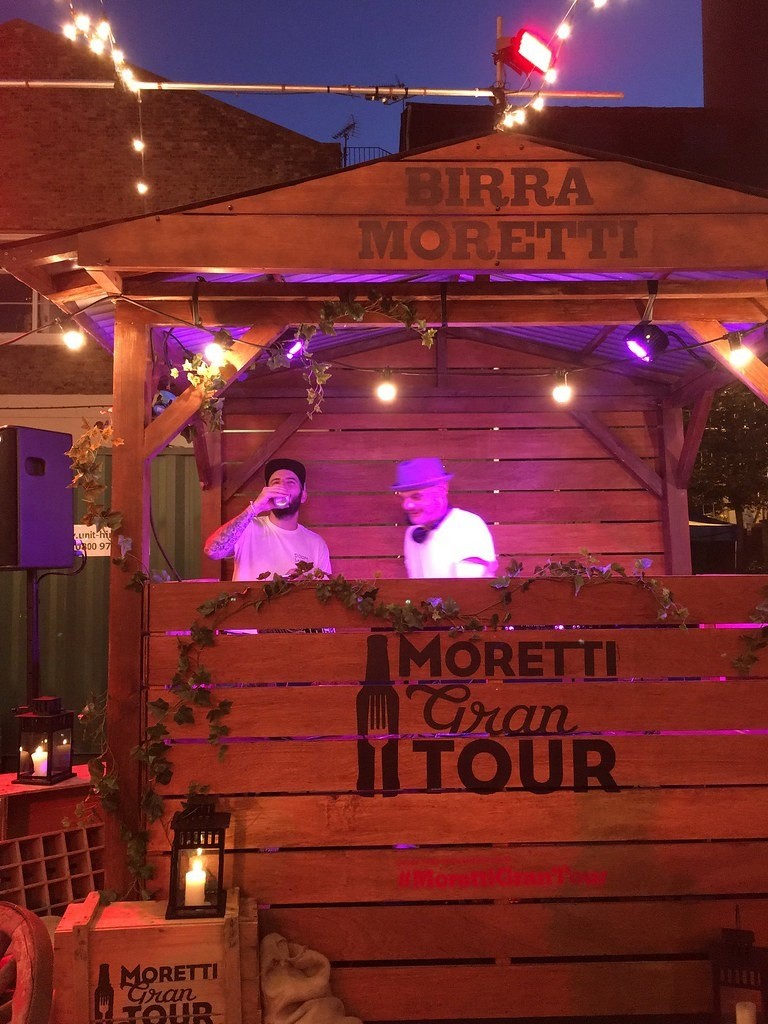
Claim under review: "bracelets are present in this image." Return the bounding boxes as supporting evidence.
[250,500,258,517]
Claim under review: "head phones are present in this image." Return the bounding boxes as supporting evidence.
[412,505,449,544]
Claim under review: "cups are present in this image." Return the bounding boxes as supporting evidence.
[273,495,290,506]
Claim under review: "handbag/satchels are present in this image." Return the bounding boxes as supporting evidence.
[260,932,362,1024]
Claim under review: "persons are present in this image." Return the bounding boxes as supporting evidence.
[204,458,336,635]
[387,458,498,580]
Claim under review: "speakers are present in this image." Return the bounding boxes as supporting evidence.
[0,425,76,569]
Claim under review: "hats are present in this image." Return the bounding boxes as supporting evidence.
[264,458,306,485]
[390,458,453,491]
